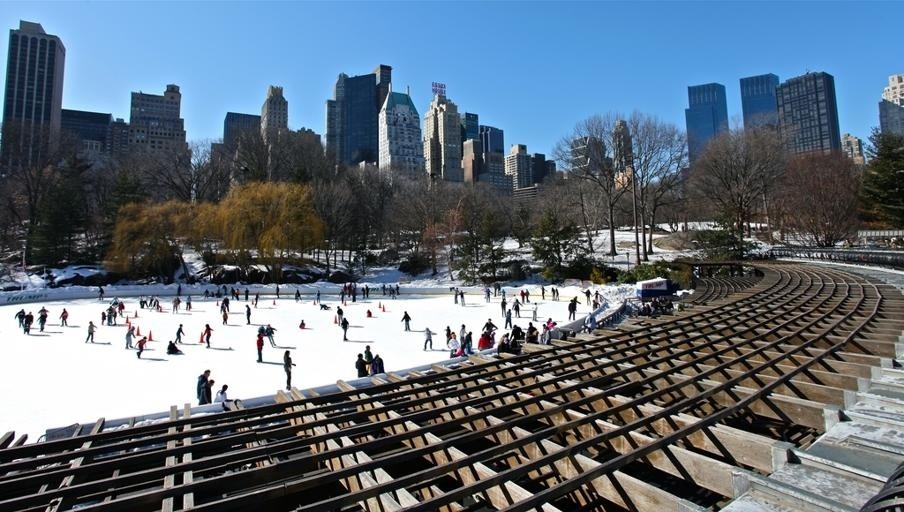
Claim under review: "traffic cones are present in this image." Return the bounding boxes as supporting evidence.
[159,306,163,312]
[124,309,154,342]
[333,315,338,325]
[198,332,207,344]
[251,299,256,305]
[272,298,277,306]
[344,300,348,306]
[378,300,387,311]
[313,300,317,306]
[215,300,220,307]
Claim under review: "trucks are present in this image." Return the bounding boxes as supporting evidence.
[635,278,681,303]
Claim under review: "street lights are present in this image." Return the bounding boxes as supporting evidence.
[21,245,26,290]
[625,252,630,271]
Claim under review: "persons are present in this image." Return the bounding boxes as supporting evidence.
[401,311,411,331]
[299,320,306,329]
[284,351,292,390]
[316,284,400,310]
[638,296,674,316]
[86,321,97,343]
[640,295,674,316]
[256,324,277,362]
[295,289,302,303]
[356,346,384,377]
[276,285,279,299]
[60,308,68,326]
[423,328,432,351]
[15,306,49,334]
[197,370,227,405]
[99,284,259,358]
[446,284,603,359]
